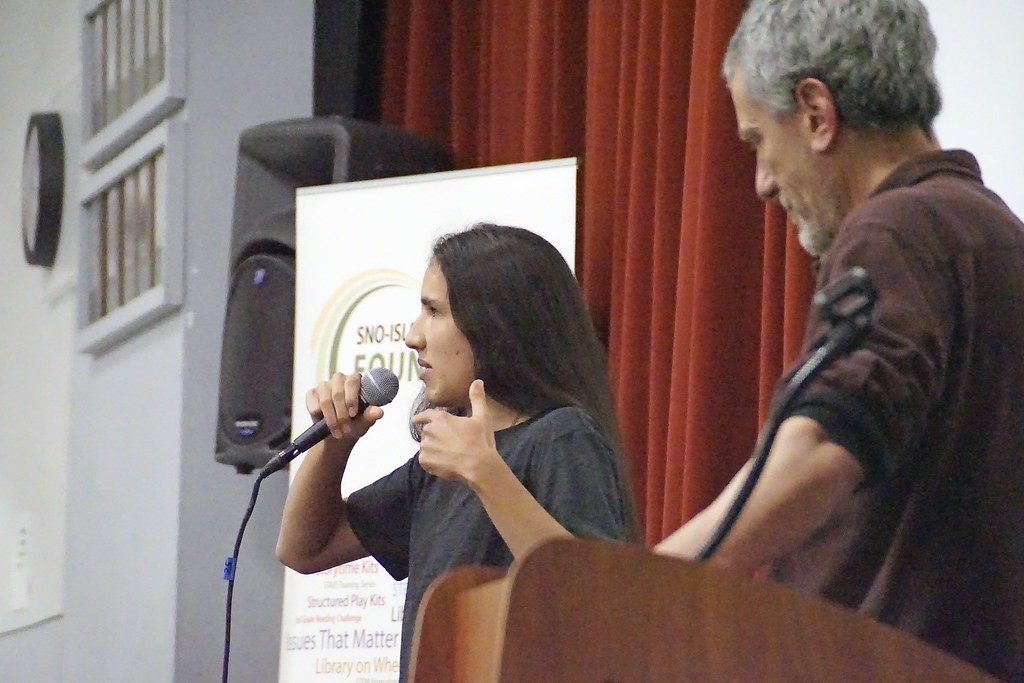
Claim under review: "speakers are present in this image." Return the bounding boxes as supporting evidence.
[213,116,454,474]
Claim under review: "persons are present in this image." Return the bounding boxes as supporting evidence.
[654,0,1024,683]
[274,220,643,683]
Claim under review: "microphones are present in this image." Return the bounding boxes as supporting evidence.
[260,366,399,481]
